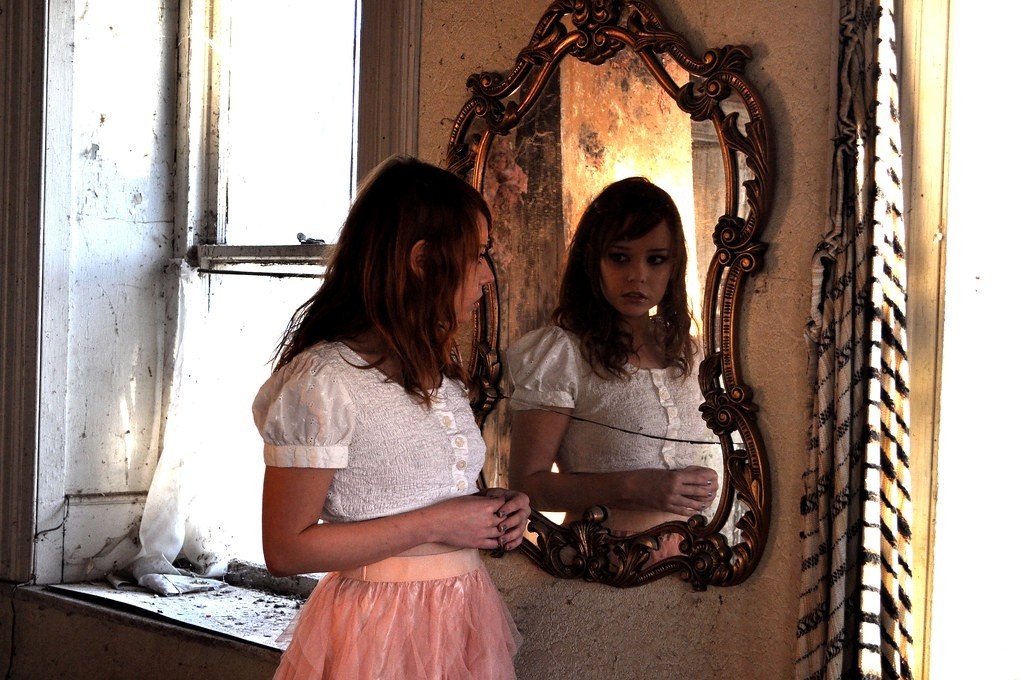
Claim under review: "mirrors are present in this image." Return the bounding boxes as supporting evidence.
[441,0,781,592]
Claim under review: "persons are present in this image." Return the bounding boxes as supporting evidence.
[252,153,531,680]
[506,176,724,567]
